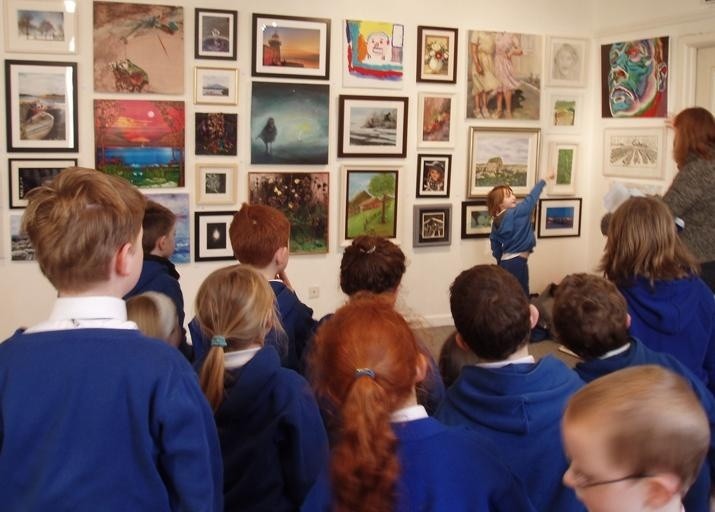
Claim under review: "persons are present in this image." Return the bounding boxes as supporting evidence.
[127,194,715,512]
[607,37,670,119]
[469,32,502,120]
[362,24,388,62]
[487,171,554,298]
[660,106,714,296]
[491,33,525,119]
[0,166,225,511]
[554,43,580,80]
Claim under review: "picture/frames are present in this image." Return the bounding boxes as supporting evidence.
[339,161,405,248]
[466,125,541,200]
[194,210,239,262]
[461,200,537,239]
[601,127,666,180]
[546,142,579,196]
[538,198,582,238]
[4,59,78,153]
[2,0,79,56]
[194,7,238,61]
[337,94,409,158]
[412,203,452,248]
[195,162,238,205]
[416,153,452,199]
[416,25,458,84]
[8,158,78,209]
[544,90,583,134]
[193,64,240,106]
[251,12,331,80]
[545,34,590,89]
[417,91,457,149]
